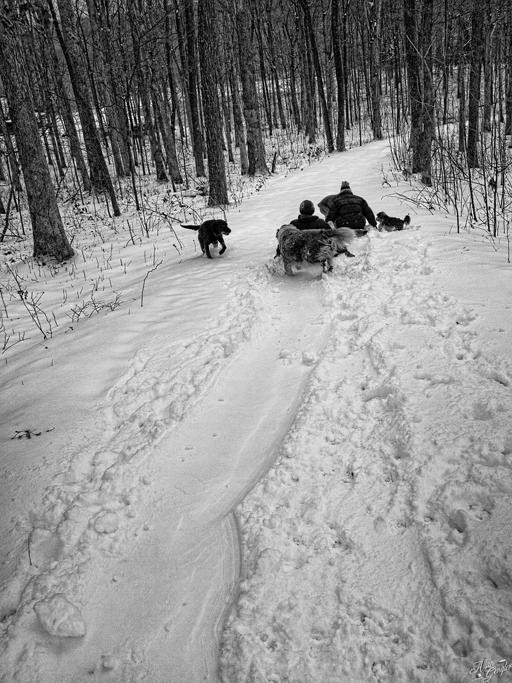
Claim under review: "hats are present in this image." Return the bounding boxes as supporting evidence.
[300,200,315,214]
[341,181,350,189]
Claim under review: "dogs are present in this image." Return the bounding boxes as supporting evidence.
[275,224,356,277]
[374,211,411,232]
[179,218,233,260]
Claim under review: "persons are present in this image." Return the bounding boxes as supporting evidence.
[325,181,377,229]
[290,200,332,229]
